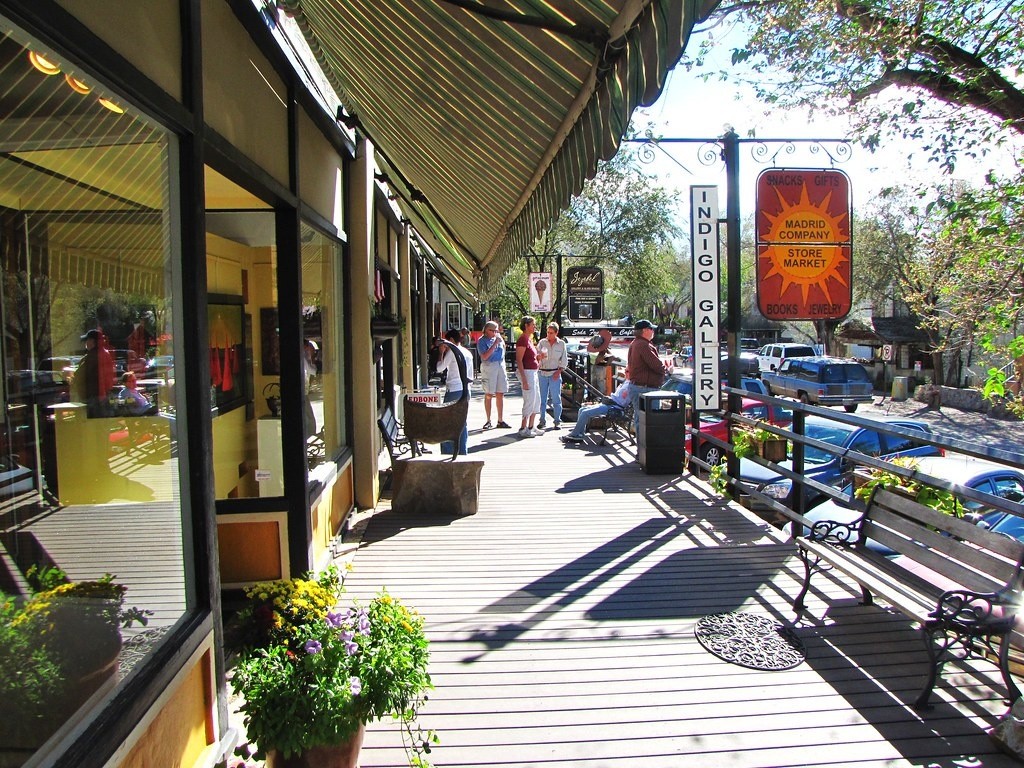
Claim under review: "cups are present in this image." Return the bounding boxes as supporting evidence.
[496,332,500,339]
[541,347,548,359]
[664,359,672,367]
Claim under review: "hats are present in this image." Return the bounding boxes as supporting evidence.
[634,320,658,329]
[79,330,105,340]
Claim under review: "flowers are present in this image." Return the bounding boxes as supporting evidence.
[243,561,427,710]
[707,420,780,490]
[0,567,124,631]
[865,453,922,480]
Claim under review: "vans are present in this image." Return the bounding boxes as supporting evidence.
[37,356,82,370]
[756,343,816,371]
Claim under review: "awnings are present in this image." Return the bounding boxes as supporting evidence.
[273,0,724,308]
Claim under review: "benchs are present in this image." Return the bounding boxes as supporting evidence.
[795,485,1024,711]
[307,426,325,461]
[377,405,432,490]
[581,402,636,446]
[120,393,169,456]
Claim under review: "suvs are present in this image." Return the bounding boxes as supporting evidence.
[716,416,945,522]
[658,373,770,415]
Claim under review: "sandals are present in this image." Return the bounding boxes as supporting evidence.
[497,422,511,428]
[483,422,491,429]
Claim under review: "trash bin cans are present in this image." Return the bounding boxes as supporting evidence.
[638,390,686,476]
[560,358,576,384]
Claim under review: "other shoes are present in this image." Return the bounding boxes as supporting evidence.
[555,424,561,430]
[562,435,584,442]
[537,423,546,428]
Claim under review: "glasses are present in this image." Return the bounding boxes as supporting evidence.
[489,329,497,333]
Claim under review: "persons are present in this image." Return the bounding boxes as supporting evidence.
[536,322,568,430]
[58,330,151,417]
[431,327,471,351]
[559,365,632,442]
[477,322,512,429]
[515,316,547,437]
[628,320,674,463]
[437,329,474,456]
[262,337,319,445]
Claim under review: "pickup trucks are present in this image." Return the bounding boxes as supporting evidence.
[761,356,875,412]
[742,338,761,354]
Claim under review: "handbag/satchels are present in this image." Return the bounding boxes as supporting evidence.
[602,396,625,410]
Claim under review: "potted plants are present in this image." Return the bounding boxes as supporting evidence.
[372,294,404,363]
[302,293,321,341]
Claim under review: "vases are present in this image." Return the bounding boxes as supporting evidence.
[732,429,787,460]
[266,715,365,768]
[0,632,121,749]
[854,468,922,503]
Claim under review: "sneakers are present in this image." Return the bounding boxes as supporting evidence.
[518,429,536,437]
[530,428,545,436]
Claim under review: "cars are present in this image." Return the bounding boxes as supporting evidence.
[684,396,794,472]
[718,352,760,378]
[782,452,1024,643]
[672,346,694,367]
[8,370,66,407]
[110,348,174,380]
